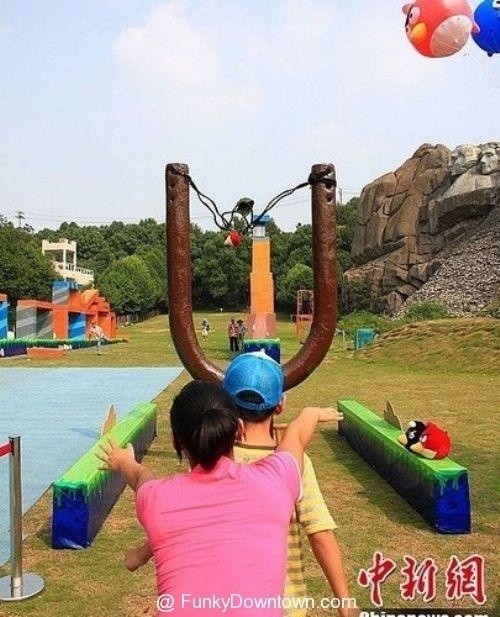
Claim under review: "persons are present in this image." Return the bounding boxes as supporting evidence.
[237,319,247,350]
[90,378,351,617]
[227,316,238,352]
[124,344,351,617]
[201,317,210,342]
[89,319,105,354]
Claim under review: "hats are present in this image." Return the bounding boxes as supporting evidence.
[221,351,284,412]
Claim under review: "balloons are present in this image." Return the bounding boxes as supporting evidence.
[402,0,482,59]
[470,1,500,57]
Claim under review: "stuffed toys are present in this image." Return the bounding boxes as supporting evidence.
[408,420,451,460]
